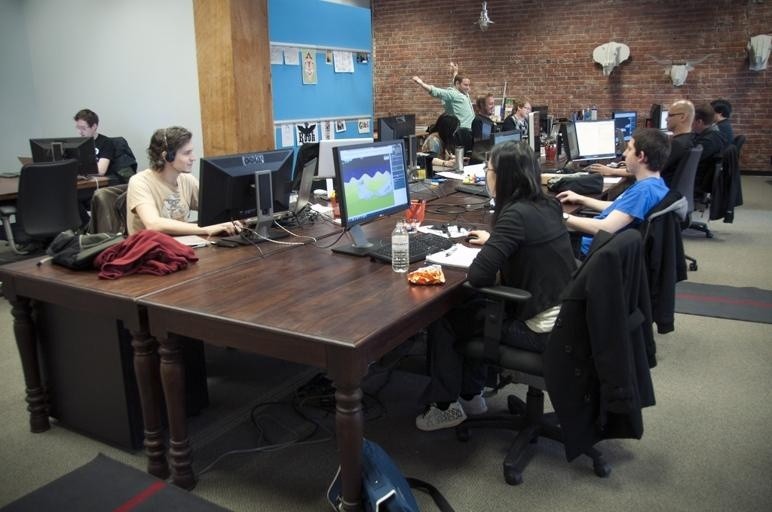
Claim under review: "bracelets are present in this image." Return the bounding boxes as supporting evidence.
[443,160,445,167]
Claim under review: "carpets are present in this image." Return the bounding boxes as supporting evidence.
[674,279,772,325]
[1,452,232,512]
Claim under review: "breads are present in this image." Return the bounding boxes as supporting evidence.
[406,263,445,285]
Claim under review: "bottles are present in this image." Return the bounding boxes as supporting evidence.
[391,222,409,273]
[454,145,465,172]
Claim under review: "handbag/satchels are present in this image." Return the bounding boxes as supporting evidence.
[327,436,419,512]
[547,173,604,194]
[48,229,126,271]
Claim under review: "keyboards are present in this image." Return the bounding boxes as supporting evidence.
[367,231,456,269]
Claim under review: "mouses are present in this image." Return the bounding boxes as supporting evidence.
[464,234,479,242]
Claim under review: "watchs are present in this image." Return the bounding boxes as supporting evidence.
[562,212,569,226]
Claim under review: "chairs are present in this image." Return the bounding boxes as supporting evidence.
[0,158,84,256]
[92,184,127,239]
[108,137,133,183]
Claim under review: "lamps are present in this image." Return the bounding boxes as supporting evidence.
[474,0,494,32]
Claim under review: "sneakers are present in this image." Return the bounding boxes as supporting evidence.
[416,389,488,431]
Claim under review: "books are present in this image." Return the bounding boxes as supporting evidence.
[527,110,541,153]
[590,108,598,121]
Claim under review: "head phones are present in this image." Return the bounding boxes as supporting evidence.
[163,128,176,162]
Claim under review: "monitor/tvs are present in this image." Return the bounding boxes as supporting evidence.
[198,146,295,248]
[532,106,548,144]
[644,103,661,129]
[557,121,580,161]
[377,113,418,184]
[330,138,411,257]
[661,110,668,130]
[612,111,638,157]
[574,119,616,167]
[29,135,99,175]
[528,110,540,159]
[489,129,522,146]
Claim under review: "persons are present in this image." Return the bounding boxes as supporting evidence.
[73,108,137,235]
[411,60,476,163]
[690,98,736,202]
[420,112,461,168]
[501,96,531,143]
[554,127,673,265]
[587,98,700,190]
[415,139,579,431]
[471,92,500,165]
[126,126,252,237]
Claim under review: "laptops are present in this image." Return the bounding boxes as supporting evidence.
[289,157,318,216]
[312,137,374,181]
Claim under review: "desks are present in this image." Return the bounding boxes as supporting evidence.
[0,179,459,455]
[373,130,429,151]
[0,172,110,201]
[137,169,627,512]
